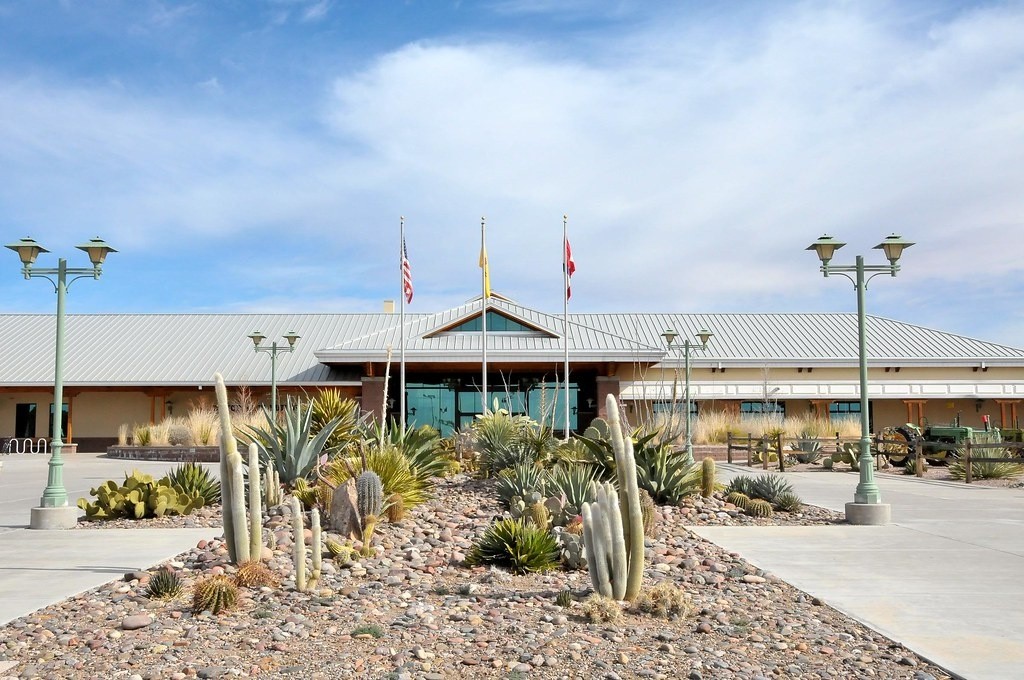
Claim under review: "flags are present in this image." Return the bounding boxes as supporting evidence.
[563,238,576,302]
[479,236,490,299]
[400,237,413,303]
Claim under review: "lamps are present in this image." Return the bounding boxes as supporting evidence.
[586,399,596,408]
[387,398,395,409]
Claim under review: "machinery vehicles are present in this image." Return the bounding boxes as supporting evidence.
[883,411,973,465]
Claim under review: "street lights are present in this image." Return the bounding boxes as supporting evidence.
[807,230,917,523]
[248,331,300,434]
[661,328,714,462]
[3,234,119,532]
